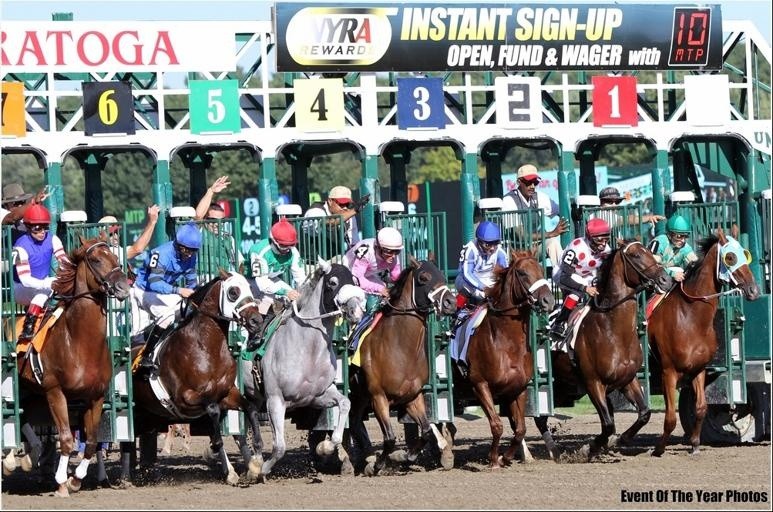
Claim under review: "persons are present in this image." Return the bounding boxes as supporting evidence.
[341,227,404,351]
[11,204,74,339]
[302,185,371,264]
[128,223,201,368]
[239,221,305,351]
[585,188,665,249]
[1,184,44,247]
[98,204,161,274]
[503,165,571,268]
[638,216,699,335]
[448,222,509,338]
[552,218,614,338]
[195,176,245,286]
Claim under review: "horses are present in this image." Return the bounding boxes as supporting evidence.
[348,253,458,477]
[519,235,670,462]
[214,255,364,487]
[645,224,760,451]
[2,231,130,496]
[120,264,264,488]
[449,245,556,468]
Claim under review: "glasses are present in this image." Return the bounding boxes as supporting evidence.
[382,248,401,257]
[6,200,27,207]
[29,225,50,233]
[518,179,540,187]
[208,217,226,225]
[590,237,610,244]
[603,199,623,208]
[335,199,353,211]
[480,240,502,249]
[671,234,689,241]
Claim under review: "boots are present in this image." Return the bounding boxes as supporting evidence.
[19,301,42,342]
[550,297,578,339]
[138,326,165,370]
[247,312,275,353]
[442,287,472,339]
[346,314,374,352]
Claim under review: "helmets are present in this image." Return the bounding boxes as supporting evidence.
[374,227,405,250]
[22,203,52,224]
[584,219,611,255]
[664,215,691,249]
[176,222,202,250]
[269,219,297,251]
[475,220,502,242]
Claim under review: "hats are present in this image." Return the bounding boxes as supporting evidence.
[599,187,626,202]
[1,183,32,204]
[516,164,542,181]
[327,186,356,205]
[97,214,123,237]
